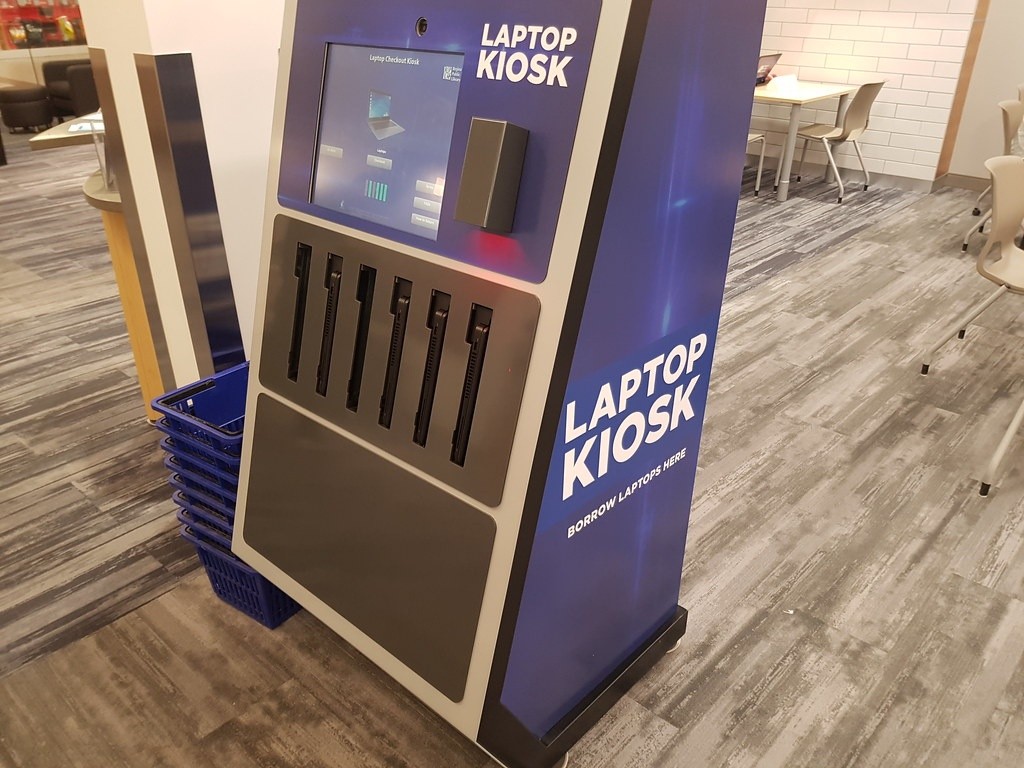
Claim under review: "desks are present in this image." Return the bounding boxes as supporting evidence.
[749,82,859,201]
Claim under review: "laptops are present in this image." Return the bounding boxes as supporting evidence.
[755,54,782,84]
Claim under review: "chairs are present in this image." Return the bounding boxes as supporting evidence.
[745,133,766,195]
[793,79,887,204]
[922,88,1024,496]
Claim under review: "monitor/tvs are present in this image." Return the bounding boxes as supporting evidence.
[312,44,465,241]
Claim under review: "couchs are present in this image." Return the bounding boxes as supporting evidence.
[43,58,98,128]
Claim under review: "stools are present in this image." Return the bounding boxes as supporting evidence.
[0,83,52,136]
